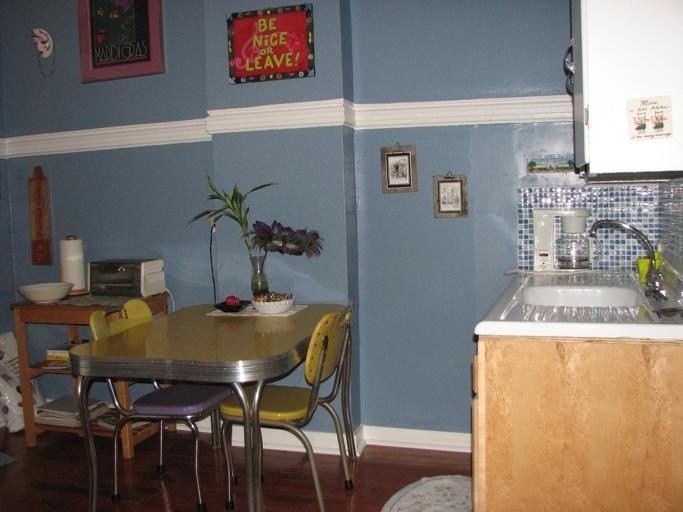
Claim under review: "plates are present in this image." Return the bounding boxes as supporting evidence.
[215,301,249,313]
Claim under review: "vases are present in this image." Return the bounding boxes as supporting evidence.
[248,257,268,294]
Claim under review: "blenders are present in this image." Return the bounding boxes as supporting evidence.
[532,208,592,273]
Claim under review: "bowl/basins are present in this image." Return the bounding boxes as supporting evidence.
[17,282,74,304]
[250,298,294,312]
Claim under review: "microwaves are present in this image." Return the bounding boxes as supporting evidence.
[88,259,165,298]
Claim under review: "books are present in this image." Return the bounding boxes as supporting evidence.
[34,393,110,428]
[96,414,153,431]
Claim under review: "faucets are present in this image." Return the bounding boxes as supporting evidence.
[589,220,666,300]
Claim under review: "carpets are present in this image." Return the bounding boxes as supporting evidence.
[382,474,471,512]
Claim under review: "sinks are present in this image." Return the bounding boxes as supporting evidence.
[519,286,643,307]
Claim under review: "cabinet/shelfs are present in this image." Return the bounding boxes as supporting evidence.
[471,327,682,512]
[565,0,681,180]
[9,288,177,458]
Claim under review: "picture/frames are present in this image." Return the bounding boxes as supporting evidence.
[77,0,164,83]
[432,176,466,219]
[381,144,417,194]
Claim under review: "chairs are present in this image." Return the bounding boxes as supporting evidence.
[90,299,235,512]
[219,312,356,511]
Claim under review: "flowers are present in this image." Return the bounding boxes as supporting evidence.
[188,173,323,281]
